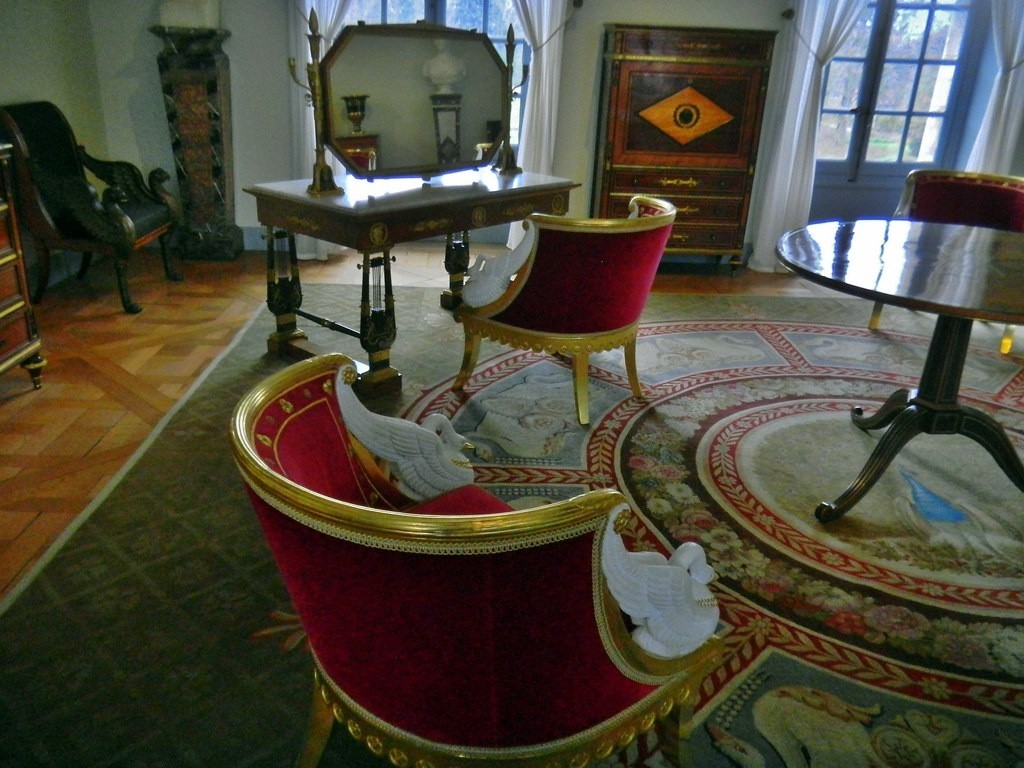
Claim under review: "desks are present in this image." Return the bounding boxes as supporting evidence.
[770,217,1024,526]
[237,162,583,403]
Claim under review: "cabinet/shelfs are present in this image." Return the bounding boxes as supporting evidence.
[0,142,53,391]
[585,18,781,276]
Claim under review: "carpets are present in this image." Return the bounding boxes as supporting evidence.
[0,281,1024,768]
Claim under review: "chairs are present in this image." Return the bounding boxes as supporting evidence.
[865,167,1024,334]
[451,191,680,428]
[222,348,730,768]
[0,98,187,315]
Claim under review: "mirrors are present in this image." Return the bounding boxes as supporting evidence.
[288,7,520,202]
